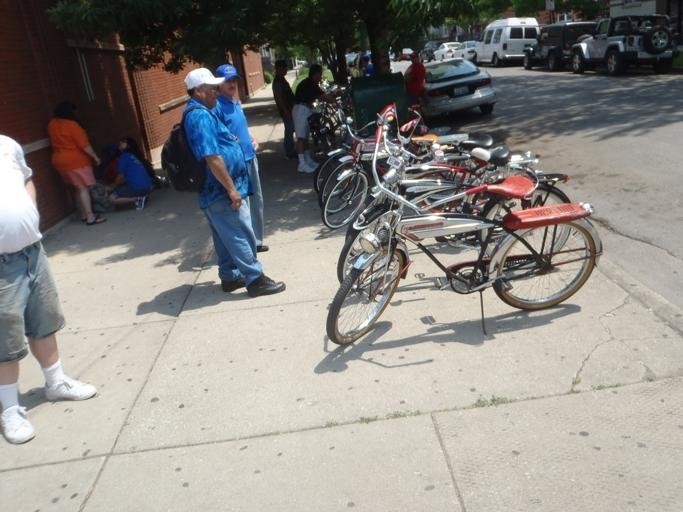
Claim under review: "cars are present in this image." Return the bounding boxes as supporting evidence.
[415,57,496,121]
[295,40,478,69]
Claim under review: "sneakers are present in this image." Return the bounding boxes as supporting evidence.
[42,377,99,402]
[0,402,36,446]
[134,195,146,210]
[285,150,300,160]
[297,159,320,174]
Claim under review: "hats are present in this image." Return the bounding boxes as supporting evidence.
[184,67,226,90]
[214,64,242,82]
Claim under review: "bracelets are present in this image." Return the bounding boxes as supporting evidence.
[94,156,98,160]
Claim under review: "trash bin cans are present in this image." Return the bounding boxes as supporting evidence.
[350,72,408,137]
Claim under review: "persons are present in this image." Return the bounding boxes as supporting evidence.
[0,135,98,445]
[404,52,427,108]
[49,102,107,226]
[274,58,296,162]
[291,65,338,174]
[212,64,270,254]
[360,54,374,75]
[106,136,157,211]
[182,67,286,297]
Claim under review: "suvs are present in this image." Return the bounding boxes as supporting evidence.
[568,14,680,72]
[523,22,598,70]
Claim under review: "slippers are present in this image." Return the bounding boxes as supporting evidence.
[80,213,108,224]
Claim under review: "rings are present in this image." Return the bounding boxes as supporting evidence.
[236,203,240,206]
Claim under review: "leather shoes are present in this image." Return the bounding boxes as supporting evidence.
[247,272,287,298]
[220,276,246,293]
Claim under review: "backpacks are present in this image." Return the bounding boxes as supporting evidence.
[159,123,208,192]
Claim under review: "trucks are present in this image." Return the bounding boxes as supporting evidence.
[471,16,540,65]
